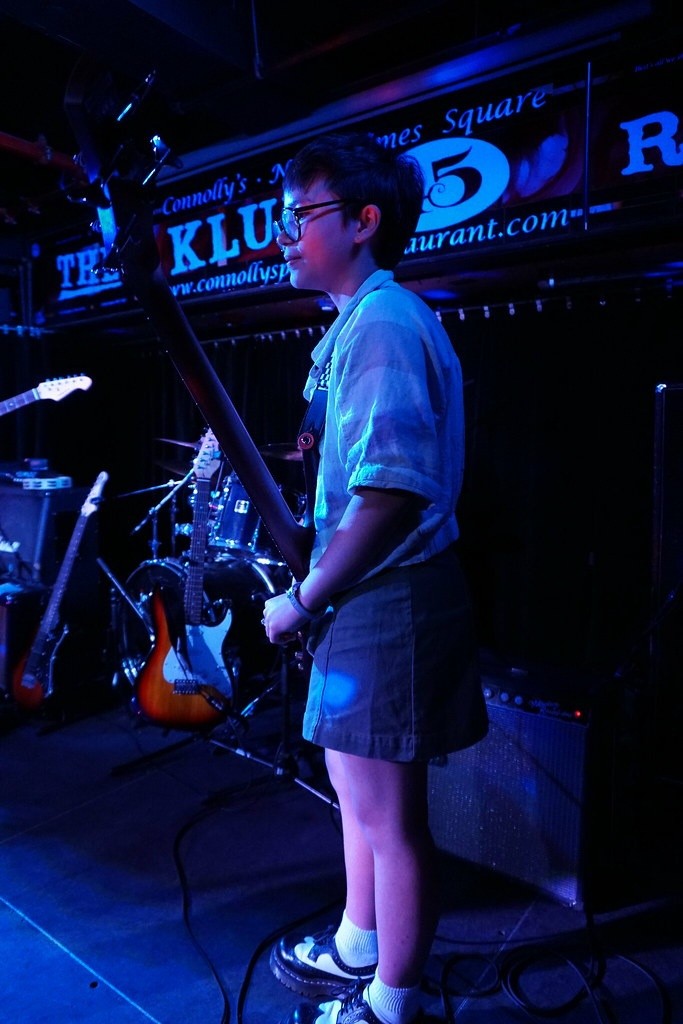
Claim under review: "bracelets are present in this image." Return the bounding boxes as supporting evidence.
[286,581,324,625]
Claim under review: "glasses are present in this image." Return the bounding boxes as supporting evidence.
[272,198,344,250]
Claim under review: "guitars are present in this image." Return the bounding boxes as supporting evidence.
[101,205,492,757]
[12,471,110,711]
[0,372,94,417]
[128,425,235,730]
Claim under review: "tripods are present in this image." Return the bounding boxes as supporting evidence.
[104,624,342,812]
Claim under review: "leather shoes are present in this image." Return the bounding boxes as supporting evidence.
[287,979,385,1024]
[269,924,377,1002]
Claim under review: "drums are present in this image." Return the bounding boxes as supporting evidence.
[118,547,298,693]
[207,472,308,567]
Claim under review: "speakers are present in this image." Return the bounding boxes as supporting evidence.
[0,483,102,589]
[418,675,657,914]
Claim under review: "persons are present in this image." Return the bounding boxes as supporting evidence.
[272,138,471,1023]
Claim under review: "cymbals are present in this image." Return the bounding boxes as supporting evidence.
[150,459,194,480]
[258,442,304,463]
[156,437,275,459]
